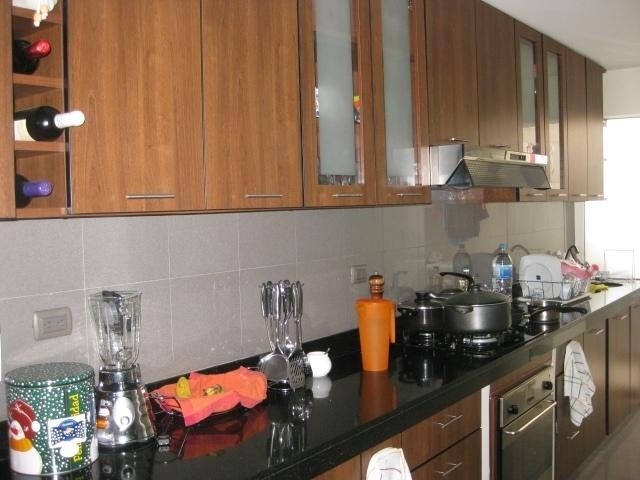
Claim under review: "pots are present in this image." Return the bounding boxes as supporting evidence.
[439,271,512,333]
[396,286,444,335]
[526,298,587,325]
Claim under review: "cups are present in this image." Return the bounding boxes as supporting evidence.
[355,298,397,371]
[305,349,331,377]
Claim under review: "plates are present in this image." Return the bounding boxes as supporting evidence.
[518,254,563,298]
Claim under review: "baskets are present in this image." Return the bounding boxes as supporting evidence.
[513,278,588,300]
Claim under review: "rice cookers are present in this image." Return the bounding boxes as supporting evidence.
[499,395,556,479]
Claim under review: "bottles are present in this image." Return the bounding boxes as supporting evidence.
[452,244,473,281]
[368,274,385,299]
[13,105,85,143]
[12,37,52,73]
[4,360,101,478]
[490,240,514,304]
[15,171,56,208]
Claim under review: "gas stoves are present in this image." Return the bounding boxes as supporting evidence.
[395,323,548,360]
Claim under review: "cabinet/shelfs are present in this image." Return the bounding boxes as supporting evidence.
[295,429,402,479]
[0,0,70,219]
[553,282,640,480]
[292,2,428,213]
[399,384,484,480]
[564,44,613,205]
[514,17,570,208]
[419,0,520,208]
[58,2,304,216]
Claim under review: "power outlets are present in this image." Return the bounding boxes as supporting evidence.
[31,302,74,344]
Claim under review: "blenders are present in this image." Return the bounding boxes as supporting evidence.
[87,289,158,450]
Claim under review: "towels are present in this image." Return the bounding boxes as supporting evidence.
[154,365,268,427]
[364,446,416,480]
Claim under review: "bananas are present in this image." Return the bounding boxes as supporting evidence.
[176,376,192,398]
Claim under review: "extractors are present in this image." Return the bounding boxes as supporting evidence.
[429,142,552,192]
